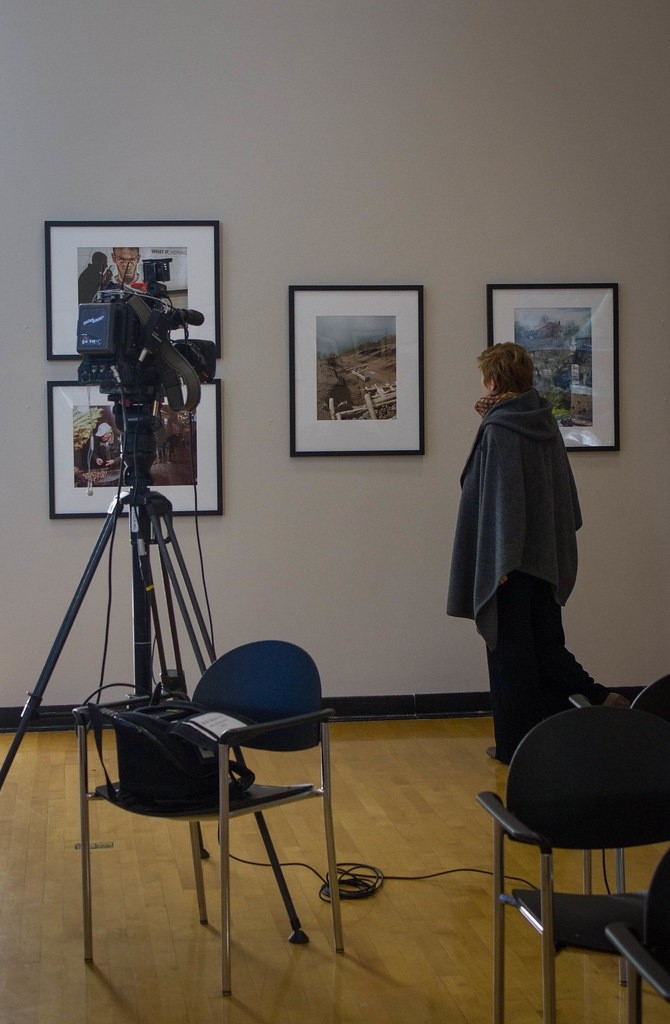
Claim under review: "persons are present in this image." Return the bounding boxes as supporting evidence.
[78,246,148,305]
[447,341,629,765]
[85,417,173,467]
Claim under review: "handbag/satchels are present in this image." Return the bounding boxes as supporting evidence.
[87,701,255,815]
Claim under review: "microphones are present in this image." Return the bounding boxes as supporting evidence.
[181,309,204,326]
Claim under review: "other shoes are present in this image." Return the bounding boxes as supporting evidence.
[602,692,630,710]
[486,747,497,759]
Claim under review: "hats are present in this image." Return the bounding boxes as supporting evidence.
[94,423,111,437]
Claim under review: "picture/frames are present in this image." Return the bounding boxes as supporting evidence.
[44,220,221,361]
[47,379,223,520]
[486,283,620,453]
[288,285,425,456]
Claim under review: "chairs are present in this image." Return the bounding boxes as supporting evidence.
[475,672,670,1024]
[71,640,344,994]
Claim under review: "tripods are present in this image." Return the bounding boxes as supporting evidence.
[0,405,309,945]
